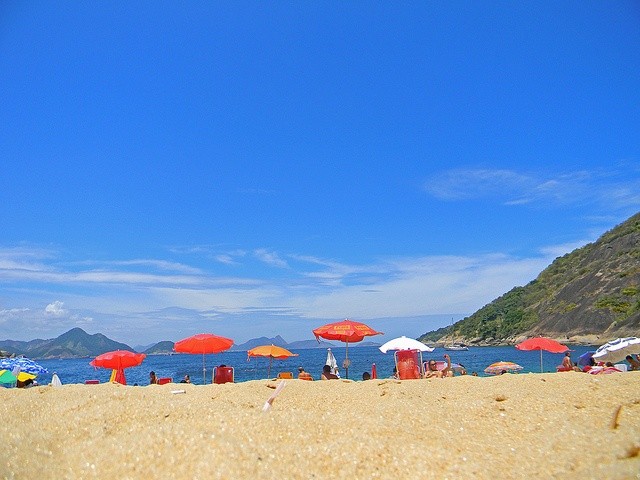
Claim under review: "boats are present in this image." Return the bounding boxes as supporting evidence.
[444,344,469,351]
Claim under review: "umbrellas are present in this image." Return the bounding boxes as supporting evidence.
[577,351,595,371]
[445,363,464,372]
[312,317,384,379]
[378,336,435,354]
[325,348,340,379]
[372,363,377,379]
[88,349,147,384]
[246,343,300,379]
[591,336,640,364]
[484,360,524,375]
[515,335,576,373]
[51,372,62,387]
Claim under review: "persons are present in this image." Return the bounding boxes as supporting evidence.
[184,374,190,384]
[561,352,572,368]
[322,365,338,379]
[625,355,640,371]
[636,354,640,361]
[174,334,234,385]
[150,371,158,384]
[298,367,314,381]
[501,370,506,375]
[458,363,466,375]
[424,354,450,379]
[363,372,370,380]
[473,371,478,377]
[214,364,232,384]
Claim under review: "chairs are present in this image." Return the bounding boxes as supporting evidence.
[84,380,101,383]
[557,366,574,372]
[299,377,314,380]
[277,372,293,379]
[158,378,172,385]
[394,349,424,379]
[212,367,235,384]
[422,361,450,377]
